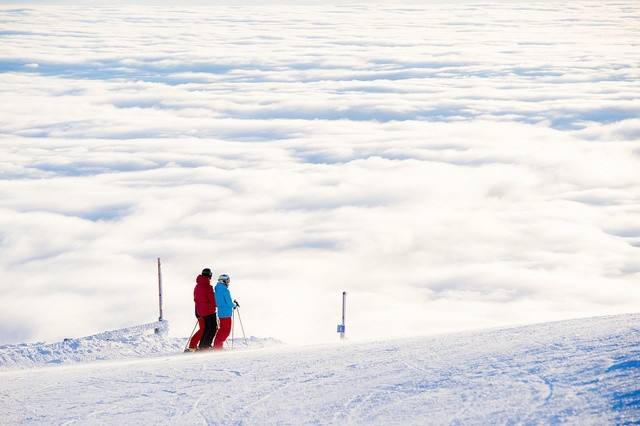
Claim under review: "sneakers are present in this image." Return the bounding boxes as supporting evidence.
[200,348,209,352]
[187,347,198,352]
[209,346,213,349]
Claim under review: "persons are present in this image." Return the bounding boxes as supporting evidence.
[194,268,217,349]
[213,274,236,351]
[189,305,205,350]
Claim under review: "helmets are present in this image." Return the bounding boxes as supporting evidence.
[202,269,212,279]
[218,275,229,284]
